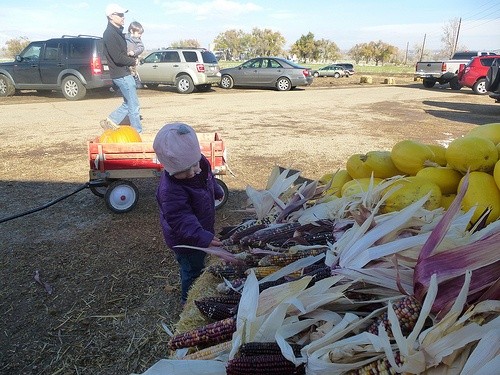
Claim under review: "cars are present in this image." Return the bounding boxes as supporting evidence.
[310,63,355,78]
[219,57,314,92]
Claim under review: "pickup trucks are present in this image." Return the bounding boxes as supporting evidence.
[413,51,498,91]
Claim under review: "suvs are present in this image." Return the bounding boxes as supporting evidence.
[457,55,500,95]
[133,47,222,95]
[0,34,118,101]
[485,57,500,104]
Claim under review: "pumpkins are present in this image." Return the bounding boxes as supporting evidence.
[320,122,500,229]
[99,119,142,143]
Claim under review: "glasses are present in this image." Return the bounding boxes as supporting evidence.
[112,13,124,17]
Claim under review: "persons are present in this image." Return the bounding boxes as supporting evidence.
[99,4,144,135]
[152,123,224,301]
[119,21,145,87]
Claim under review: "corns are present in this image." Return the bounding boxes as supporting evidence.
[169,215,422,375]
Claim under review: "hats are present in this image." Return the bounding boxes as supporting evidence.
[104,3,128,17]
[153,123,201,176]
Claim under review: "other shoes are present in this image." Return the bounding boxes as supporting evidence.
[99,119,113,131]
